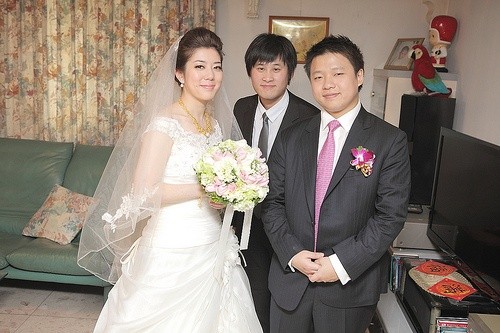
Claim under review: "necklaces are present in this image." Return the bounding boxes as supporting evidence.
[177,96,214,135]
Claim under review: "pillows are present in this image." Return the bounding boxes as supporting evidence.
[23,183,94,245]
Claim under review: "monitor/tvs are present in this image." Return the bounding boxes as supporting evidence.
[425,125,500,309]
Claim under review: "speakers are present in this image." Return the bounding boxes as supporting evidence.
[400,93,455,208]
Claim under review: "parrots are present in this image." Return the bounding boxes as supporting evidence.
[407,45,453,98]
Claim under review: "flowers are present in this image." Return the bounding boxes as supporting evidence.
[350,146,376,178]
[192,138,270,212]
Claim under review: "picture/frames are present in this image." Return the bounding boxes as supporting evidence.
[384,37,425,70]
[269,16,330,64]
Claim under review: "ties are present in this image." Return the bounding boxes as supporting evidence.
[314,120,341,253]
[258,112,269,163]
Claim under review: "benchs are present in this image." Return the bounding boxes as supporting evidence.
[0,137,114,306]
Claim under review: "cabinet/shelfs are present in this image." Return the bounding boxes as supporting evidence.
[365,246,500,333]
[370,69,458,128]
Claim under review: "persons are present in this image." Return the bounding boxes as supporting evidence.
[427,14,457,73]
[392,46,409,64]
[227,34,319,333]
[257,34,412,333]
[76,28,265,333]
